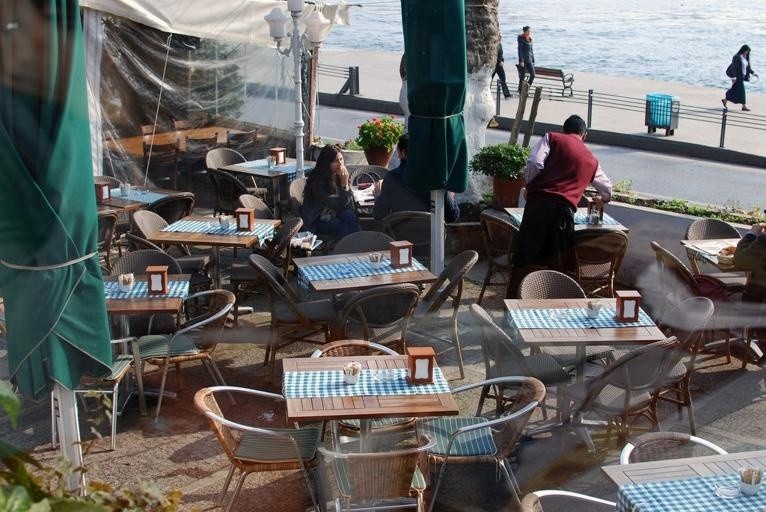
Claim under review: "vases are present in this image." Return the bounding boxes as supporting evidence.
[363,145,394,166]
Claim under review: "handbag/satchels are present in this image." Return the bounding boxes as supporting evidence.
[726,64,732,78]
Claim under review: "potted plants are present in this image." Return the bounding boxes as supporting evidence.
[470,144,532,207]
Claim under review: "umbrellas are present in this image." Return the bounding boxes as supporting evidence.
[0,0,114,496]
[401,0,469,277]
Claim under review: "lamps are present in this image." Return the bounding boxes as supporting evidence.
[263,0,333,57]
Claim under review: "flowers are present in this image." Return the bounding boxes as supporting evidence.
[355,114,402,148]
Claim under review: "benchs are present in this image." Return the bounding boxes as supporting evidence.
[517,62,574,91]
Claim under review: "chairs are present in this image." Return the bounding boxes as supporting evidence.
[565,337,675,444]
[311,339,415,438]
[469,303,571,428]
[521,490,616,512]
[649,218,765,373]
[573,228,629,299]
[316,431,437,512]
[193,385,321,512]
[476,209,520,306]
[333,161,478,378]
[621,430,728,466]
[657,296,715,436]
[415,375,546,511]
[519,269,587,356]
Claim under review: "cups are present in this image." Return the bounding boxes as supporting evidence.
[267,161,276,167]
[119,284,134,292]
[740,483,760,496]
[121,188,131,196]
[586,310,600,318]
[219,224,229,230]
[370,262,382,270]
[343,374,359,385]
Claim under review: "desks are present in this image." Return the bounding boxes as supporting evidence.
[600,450,762,511]
[503,297,661,456]
[282,356,458,453]
[480,198,630,233]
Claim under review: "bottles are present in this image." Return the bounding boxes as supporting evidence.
[589,209,598,225]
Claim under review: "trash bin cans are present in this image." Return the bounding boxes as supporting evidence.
[645,92,680,136]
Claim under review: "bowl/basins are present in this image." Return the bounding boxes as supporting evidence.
[717,255,734,265]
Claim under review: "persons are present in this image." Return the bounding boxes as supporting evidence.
[505,113,611,313]
[372,132,460,224]
[301,143,361,238]
[732,222,766,304]
[492,34,514,98]
[516,26,535,94]
[722,45,759,112]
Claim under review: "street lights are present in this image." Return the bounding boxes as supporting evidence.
[264,1,329,181]
